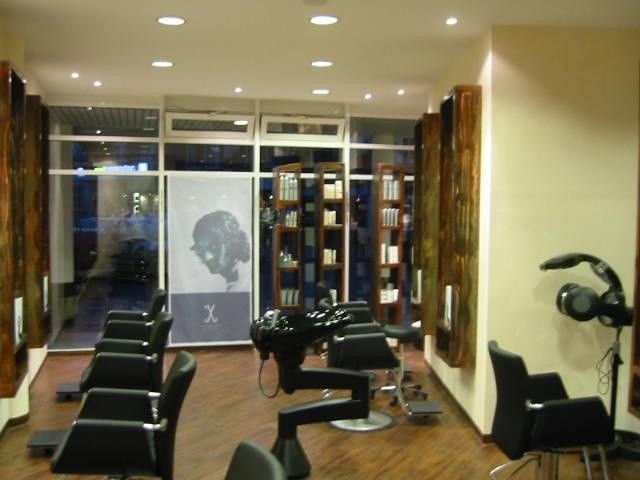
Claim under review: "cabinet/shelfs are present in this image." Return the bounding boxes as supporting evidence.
[271,162,405,354]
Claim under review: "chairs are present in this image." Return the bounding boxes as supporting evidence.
[222,437,288,479]
[50,350,197,477]
[485,339,614,480]
[27,312,176,448]
[328,322,442,431]
[55,287,167,396]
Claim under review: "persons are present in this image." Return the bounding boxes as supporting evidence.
[189,210,250,293]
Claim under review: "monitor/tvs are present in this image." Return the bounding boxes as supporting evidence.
[71,159,154,232]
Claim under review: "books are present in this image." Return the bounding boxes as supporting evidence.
[278,178,301,308]
[377,179,401,305]
[322,178,342,266]
